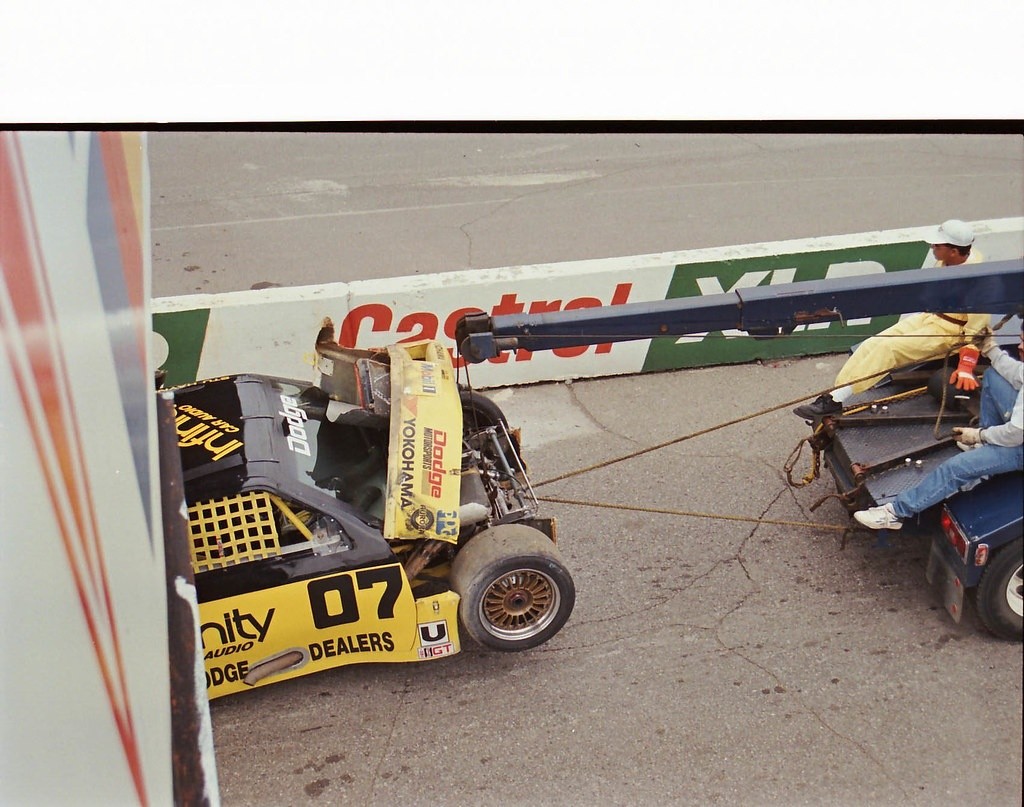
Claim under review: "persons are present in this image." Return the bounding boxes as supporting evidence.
[793,219,992,420]
[853,321,1024,530]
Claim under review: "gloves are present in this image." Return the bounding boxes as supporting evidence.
[949,347,981,391]
[972,327,999,355]
[952,427,984,445]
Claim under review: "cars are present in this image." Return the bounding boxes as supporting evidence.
[155,316,577,703]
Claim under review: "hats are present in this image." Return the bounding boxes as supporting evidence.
[924,219,975,247]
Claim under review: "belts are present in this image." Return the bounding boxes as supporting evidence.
[933,312,968,326]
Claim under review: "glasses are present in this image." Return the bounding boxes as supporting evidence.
[931,243,947,250]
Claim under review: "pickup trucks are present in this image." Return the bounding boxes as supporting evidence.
[811,336,1024,644]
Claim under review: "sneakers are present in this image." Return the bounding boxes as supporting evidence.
[956,440,984,452]
[853,502,905,530]
[793,394,842,420]
